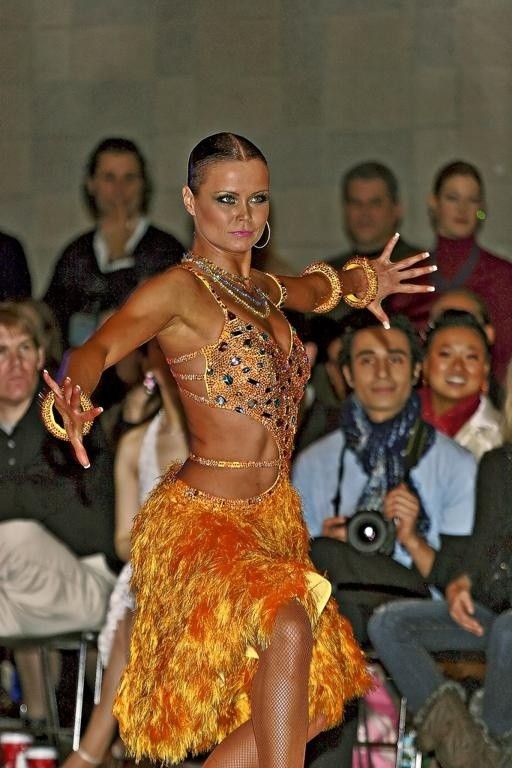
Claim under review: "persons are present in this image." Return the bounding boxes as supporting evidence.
[296,163,511,766]
[41,133,438,768]
[1,139,193,767]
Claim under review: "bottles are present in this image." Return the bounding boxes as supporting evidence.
[27,746,59,768]
[0,733,31,768]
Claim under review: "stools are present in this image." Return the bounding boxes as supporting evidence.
[1,635,105,751]
[329,579,492,767]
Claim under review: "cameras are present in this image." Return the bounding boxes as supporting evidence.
[345,509,395,557]
